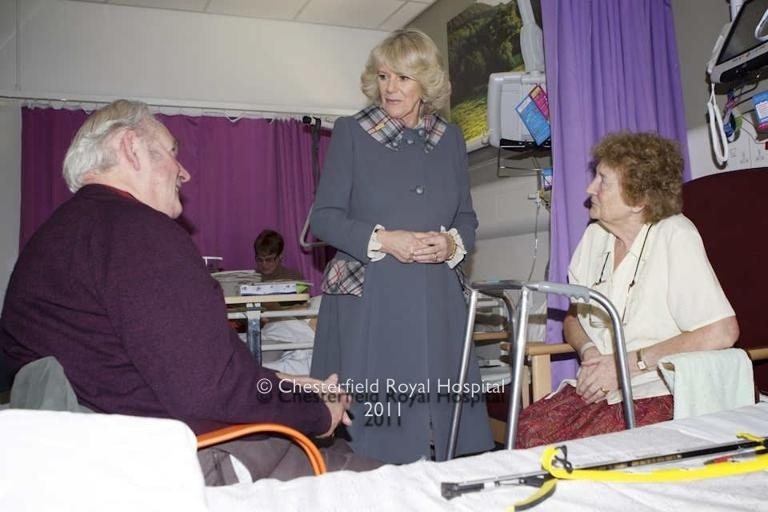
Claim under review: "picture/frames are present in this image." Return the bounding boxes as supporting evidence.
[442,1,542,153]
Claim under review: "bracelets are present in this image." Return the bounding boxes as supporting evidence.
[446,234,456,261]
[579,342,596,357]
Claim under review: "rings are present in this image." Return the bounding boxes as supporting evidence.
[600,386,609,394]
[434,253,438,261]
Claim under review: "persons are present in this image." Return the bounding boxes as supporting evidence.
[1,99,360,485]
[310,29,497,467]
[227,228,309,326]
[517,132,739,447]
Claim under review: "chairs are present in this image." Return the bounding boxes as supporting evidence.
[503,166,767,404]
[14,354,326,481]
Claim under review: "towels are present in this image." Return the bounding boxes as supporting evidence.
[659,349,753,422]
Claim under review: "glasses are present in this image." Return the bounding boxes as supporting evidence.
[255,253,279,262]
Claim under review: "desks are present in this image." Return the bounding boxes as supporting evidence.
[224,293,310,311]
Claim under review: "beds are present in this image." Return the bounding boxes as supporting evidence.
[226,295,322,374]
[0,403,768,512]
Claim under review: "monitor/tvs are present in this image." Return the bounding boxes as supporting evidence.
[709,0,768,86]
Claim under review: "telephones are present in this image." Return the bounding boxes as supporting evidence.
[708,22,732,73]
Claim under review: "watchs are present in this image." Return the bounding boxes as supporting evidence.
[634,349,650,375]
